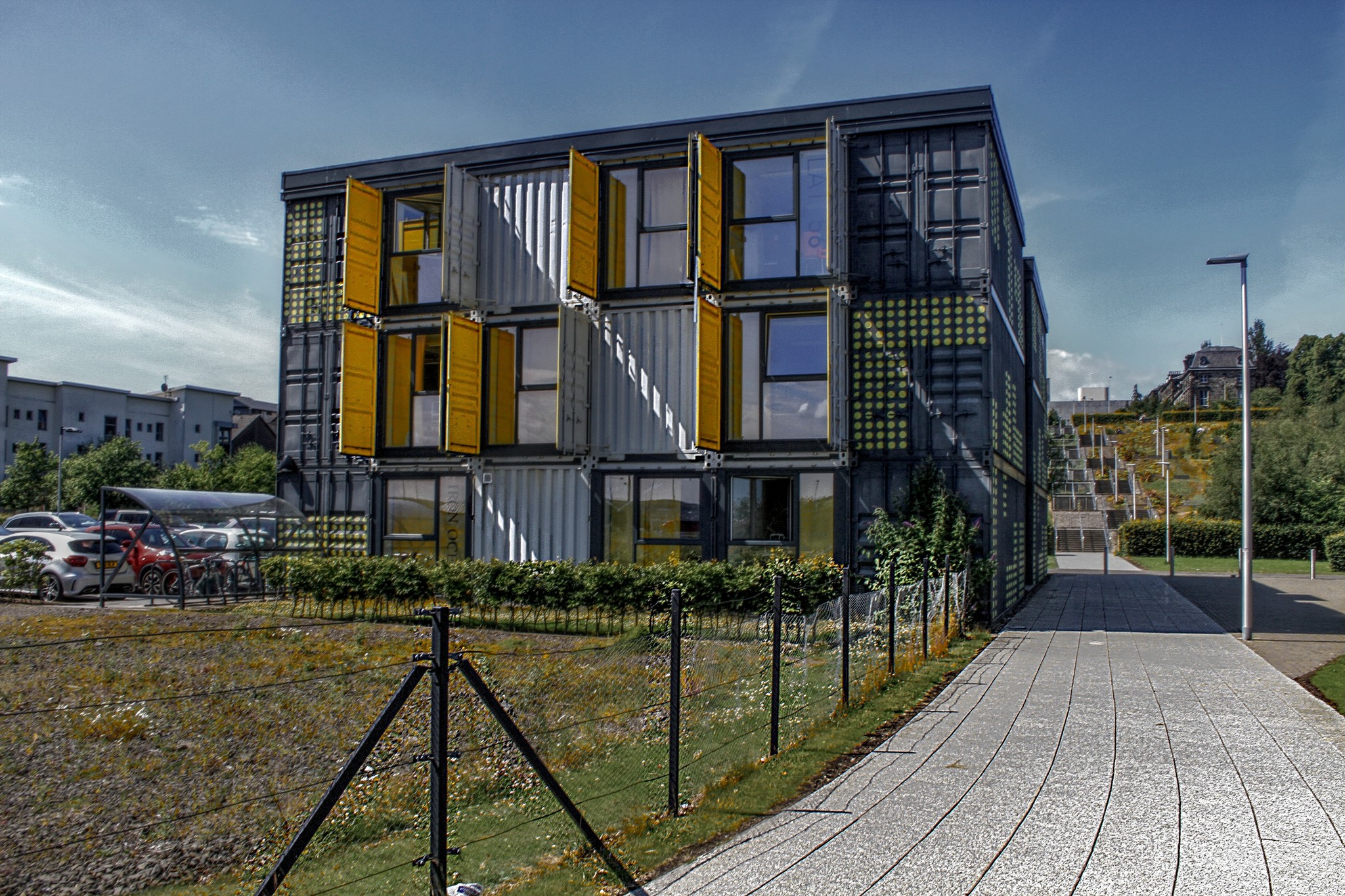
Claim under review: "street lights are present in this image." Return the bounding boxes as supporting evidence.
[1096,426,1104,478]
[1153,414,1160,457]
[57,425,82,510]
[1108,440,1120,504]
[1205,251,1254,643]
[1156,461,1173,564]
[1125,462,1137,522]
[1088,413,1096,458]
[1162,426,1167,477]
[1081,395,1093,431]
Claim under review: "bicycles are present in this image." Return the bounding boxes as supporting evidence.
[160,544,284,604]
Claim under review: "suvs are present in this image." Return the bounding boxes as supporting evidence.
[84,522,230,597]
[0,512,100,536]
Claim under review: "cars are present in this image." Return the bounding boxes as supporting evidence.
[0,531,137,603]
[107,509,281,543]
[160,527,273,581]
